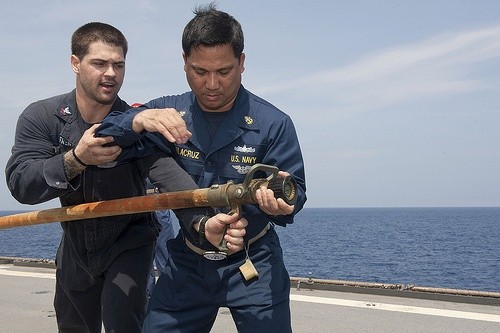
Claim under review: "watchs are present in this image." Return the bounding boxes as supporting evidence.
[199,216,210,244]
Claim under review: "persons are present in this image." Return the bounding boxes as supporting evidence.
[94,1,307,333]
[6,21,248,333]
